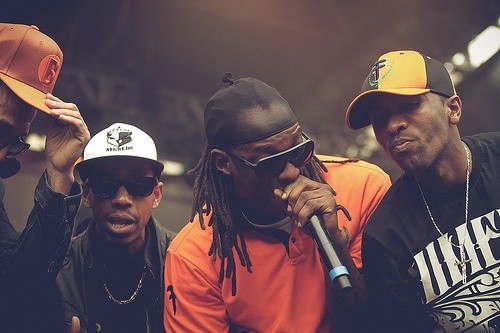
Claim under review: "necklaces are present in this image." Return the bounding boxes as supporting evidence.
[413,140,473,284]
[101,272,147,305]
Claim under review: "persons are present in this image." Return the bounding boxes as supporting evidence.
[345,49,500,333]
[163,75,393,333]
[0,21,92,331]
[55,121,181,333]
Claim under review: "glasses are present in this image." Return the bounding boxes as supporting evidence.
[0,120,30,158]
[82,173,161,199]
[222,132,315,183]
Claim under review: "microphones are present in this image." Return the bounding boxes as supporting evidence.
[307,212,352,292]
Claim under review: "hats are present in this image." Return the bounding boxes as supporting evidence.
[205,72,298,150]
[73,122,165,183]
[346,50,457,131]
[0,23,64,120]
